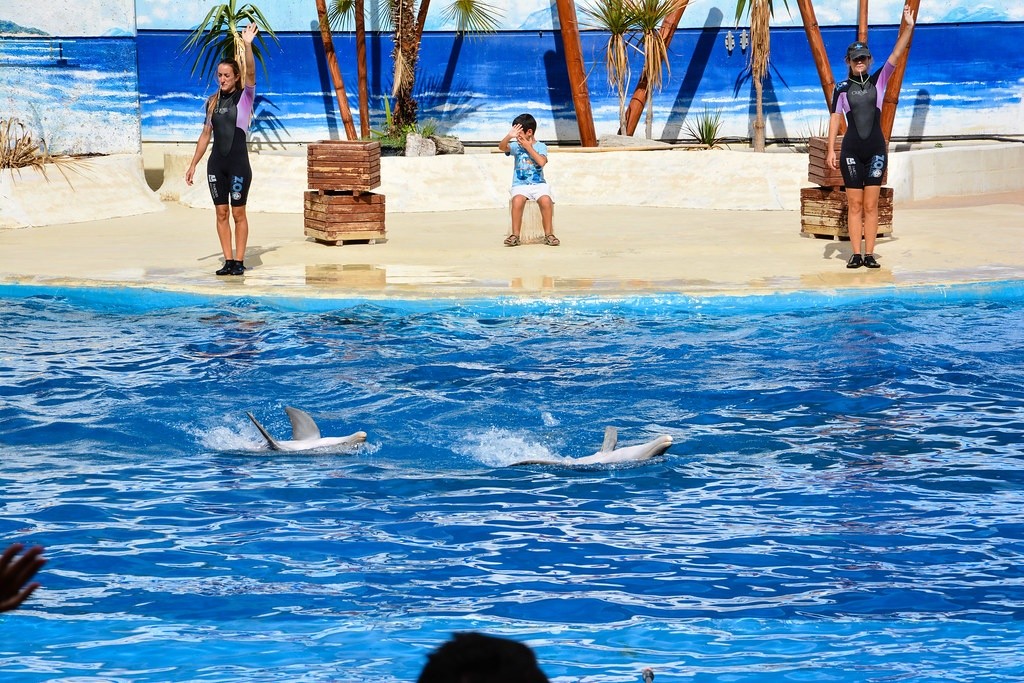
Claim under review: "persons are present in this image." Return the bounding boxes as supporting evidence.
[498,113,561,247]
[825,3,915,269]
[185,22,259,278]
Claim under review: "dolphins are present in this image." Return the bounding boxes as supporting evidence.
[510,427,674,466]
[247,407,366,452]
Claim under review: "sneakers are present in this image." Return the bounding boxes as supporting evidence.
[864,254,882,269]
[231,261,246,276]
[846,254,863,269]
[216,259,234,275]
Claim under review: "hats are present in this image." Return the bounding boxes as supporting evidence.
[846,41,871,61]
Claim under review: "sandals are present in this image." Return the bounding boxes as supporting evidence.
[504,233,520,248]
[545,234,560,246]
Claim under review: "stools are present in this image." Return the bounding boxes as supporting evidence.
[507,189,554,244]
